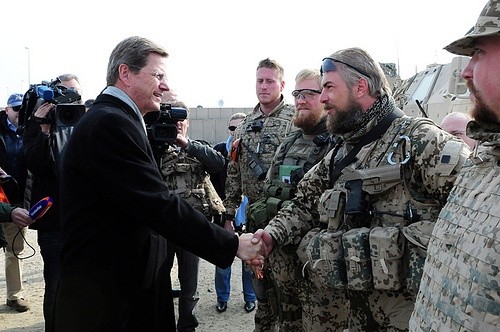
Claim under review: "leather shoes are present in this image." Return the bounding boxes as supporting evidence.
[245,301,255,311]
[217,302,227,312]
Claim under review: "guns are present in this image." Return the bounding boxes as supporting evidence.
[345,162,403,215]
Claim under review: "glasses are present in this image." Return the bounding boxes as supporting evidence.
[12,105,21,111]
[128,65,166,81]
[320,58,371,78]
[292,89,321,98]
[228,126,236,131]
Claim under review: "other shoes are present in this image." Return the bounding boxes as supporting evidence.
[6,298,28,311]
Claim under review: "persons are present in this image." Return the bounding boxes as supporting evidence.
[42,37,268,332]
[246,47,472,332]
[409,0,500,331]
[263,69,342,332]
[441,113,476,150]
[157,102,226,332]
[223,58,297,332]
[0,74,82,312]
[213,113,257,312]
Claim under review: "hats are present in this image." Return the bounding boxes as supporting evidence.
[7,94,23,107]
[444,0,500,56]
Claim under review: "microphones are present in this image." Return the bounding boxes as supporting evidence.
[30,197,53,220]
